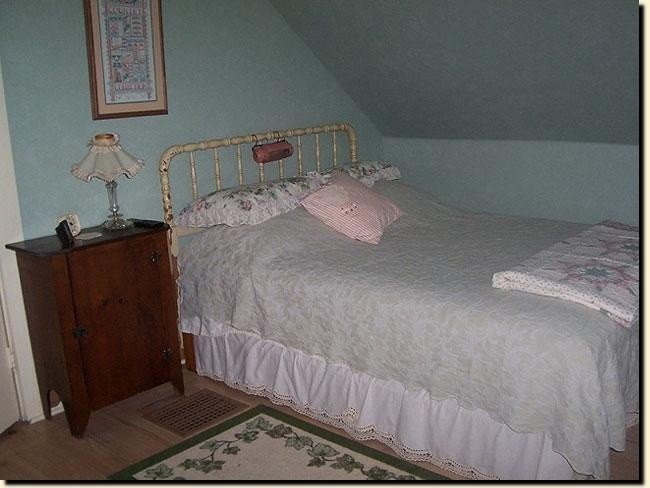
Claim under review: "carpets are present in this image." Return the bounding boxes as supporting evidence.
[104,402,452,480]
[141,388,250,439]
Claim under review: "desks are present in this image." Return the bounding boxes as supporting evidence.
[4,217,185,440]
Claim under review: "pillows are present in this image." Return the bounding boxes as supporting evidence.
[172,173,321,227]
[307,158,404,189]
[299,169,406,246]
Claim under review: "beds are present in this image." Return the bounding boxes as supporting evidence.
[158,122,640,481]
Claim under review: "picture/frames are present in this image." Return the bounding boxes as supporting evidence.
[82,0,169,121]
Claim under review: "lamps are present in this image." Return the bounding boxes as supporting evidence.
[69,132,147,231]
